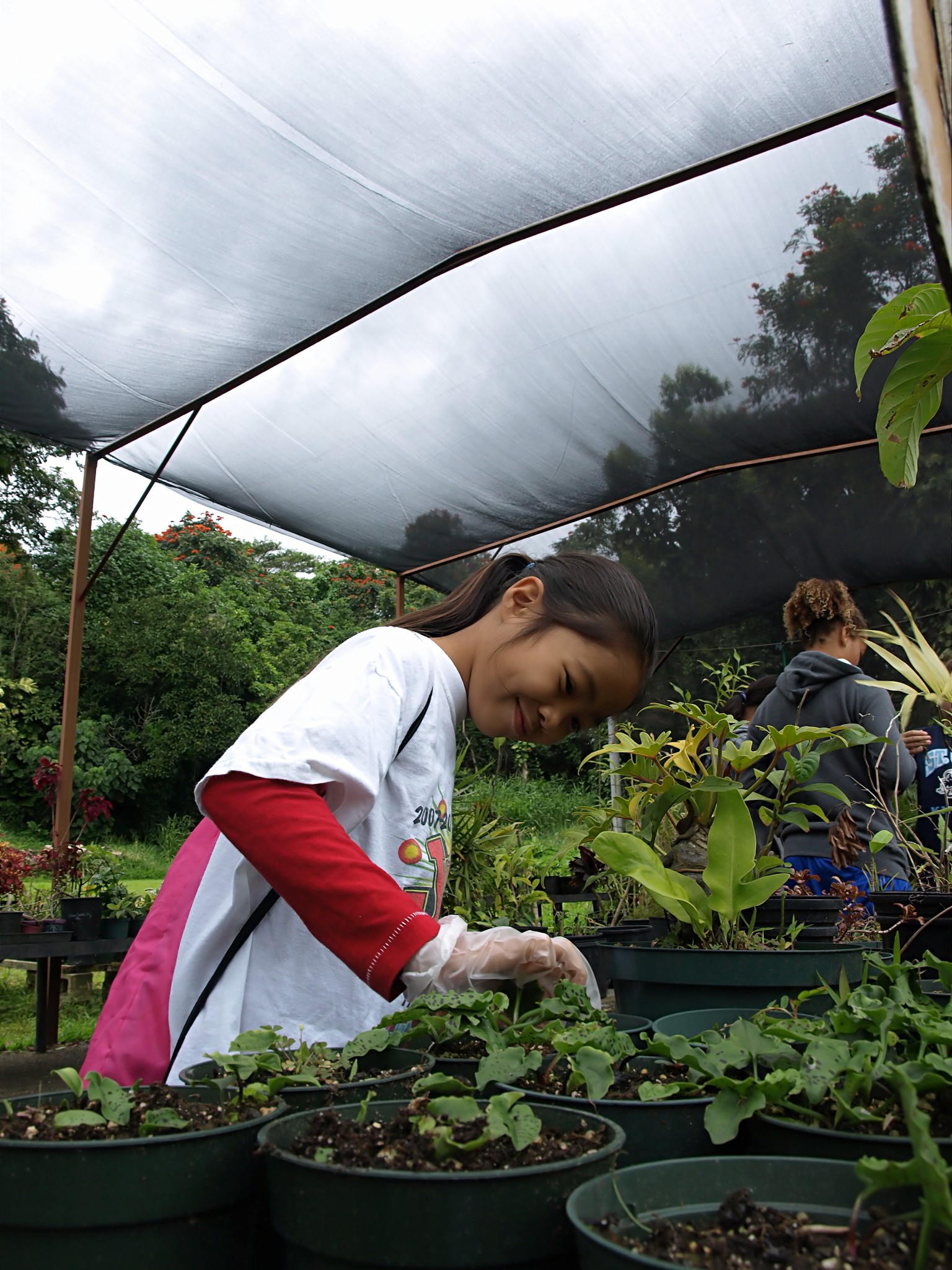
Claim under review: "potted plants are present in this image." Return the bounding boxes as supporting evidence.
[0,940,952,1269]
[0,758,147,940]
[548,651,952,1016]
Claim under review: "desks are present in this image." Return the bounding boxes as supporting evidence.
[0,938,136,1052]
[538,893,614,926]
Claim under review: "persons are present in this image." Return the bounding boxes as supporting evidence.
[692,674,778,781]
[742,579,916,921]
[77,554,659,1092]
[900,659,952,891]
